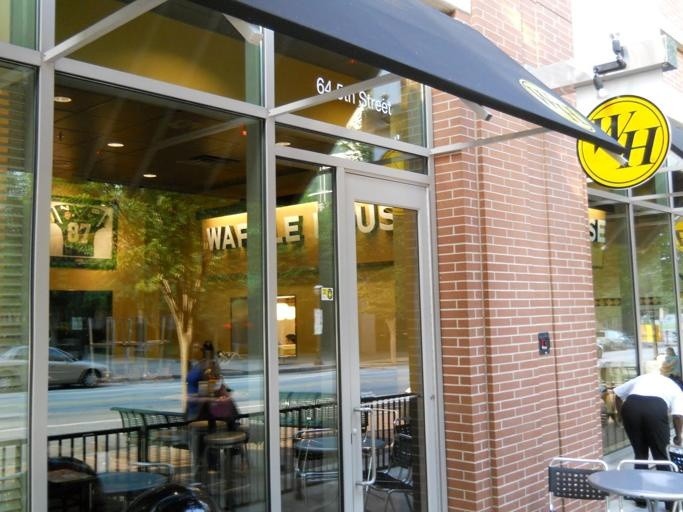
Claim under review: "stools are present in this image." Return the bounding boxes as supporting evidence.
[200,431,255,509]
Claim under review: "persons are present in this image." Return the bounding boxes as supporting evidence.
[186,342,239,465]
[613,372,683,510]
[660,348,681,381]
[286,334,296,344]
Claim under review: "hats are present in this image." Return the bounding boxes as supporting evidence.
[200,341,214,350]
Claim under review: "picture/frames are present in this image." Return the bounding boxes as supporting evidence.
[49,196,118,270]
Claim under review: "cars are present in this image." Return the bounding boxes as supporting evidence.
[596,329,631,359]
[0,344,113,390]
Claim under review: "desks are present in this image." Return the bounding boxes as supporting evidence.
[298,436,385,511]
[589,467,683,512]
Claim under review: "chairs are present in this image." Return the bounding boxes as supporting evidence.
[546,456,679,512]
[364,433,415,511]
